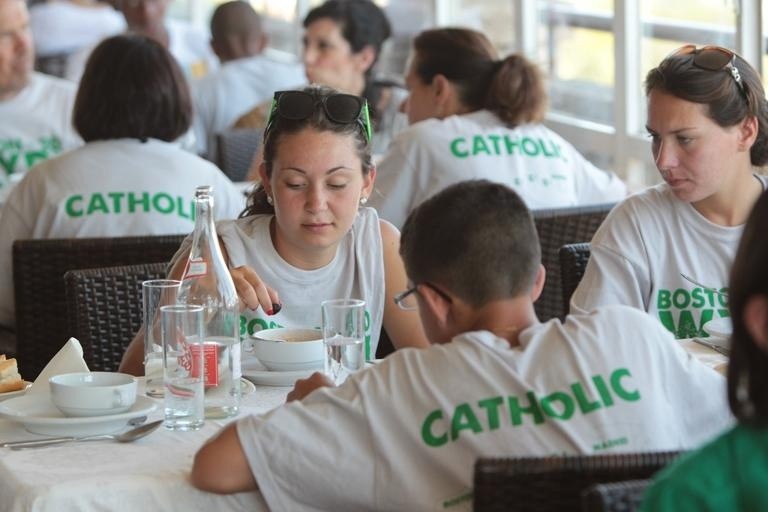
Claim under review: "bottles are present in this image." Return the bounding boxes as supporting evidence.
[175,184,243,422]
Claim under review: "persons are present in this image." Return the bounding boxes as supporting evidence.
[360,28,629,233]
[114,85,439,374]
[634,182,768,512]
[190,180,737,512]
[1,2,408,353]
[565,42,767,341]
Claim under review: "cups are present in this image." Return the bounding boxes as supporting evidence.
[1,381,34,402]
[142,279,183,398]
[159,305,207,432]
[321,299,365,388]
[49,372,138,417]
[252,329,339,371]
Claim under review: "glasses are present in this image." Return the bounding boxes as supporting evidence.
[263,90,372,143]
[392,281,452,311]
[664,45,745,96]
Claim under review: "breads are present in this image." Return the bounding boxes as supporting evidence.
[1,353,26,393]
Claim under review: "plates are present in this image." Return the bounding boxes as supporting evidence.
[703,315,734,338]
[1,394,159,437]
[242,367,330,388]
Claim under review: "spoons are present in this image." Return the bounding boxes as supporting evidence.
[1,413,165,451]
